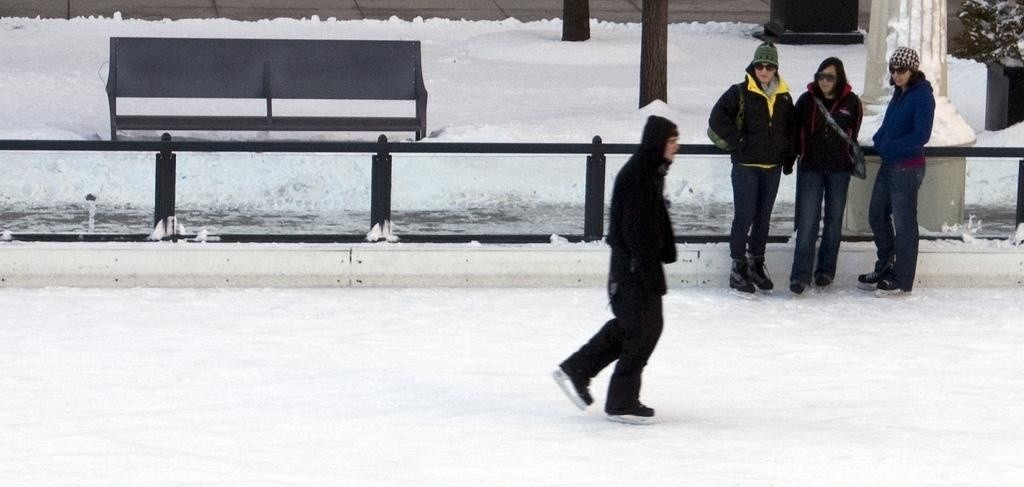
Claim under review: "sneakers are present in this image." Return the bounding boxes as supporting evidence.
[814,272,834,286]
[553,361,595,410]
[857,264,896,290]
[789,277,807,293]
[875,279,912,296]
[604,401,656,425]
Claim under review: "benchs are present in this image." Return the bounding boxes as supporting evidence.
[108,36,428,139]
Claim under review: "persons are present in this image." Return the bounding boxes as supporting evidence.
[783,57,863,295]
[706,39,794,293]
[858,47,936,291]
[559,115,680,416]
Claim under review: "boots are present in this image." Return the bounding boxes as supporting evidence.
[747,253,773,290]
[730,257,755,293]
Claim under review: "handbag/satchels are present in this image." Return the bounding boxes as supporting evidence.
[707,83,742,150]
[847,139,865,181]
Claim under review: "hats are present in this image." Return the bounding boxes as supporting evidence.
[752,41,779,68]
[889,47,920,72]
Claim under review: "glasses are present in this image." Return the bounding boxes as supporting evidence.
[754,63,777,70]
[816,72,836,82]
[888,66,909,75]
